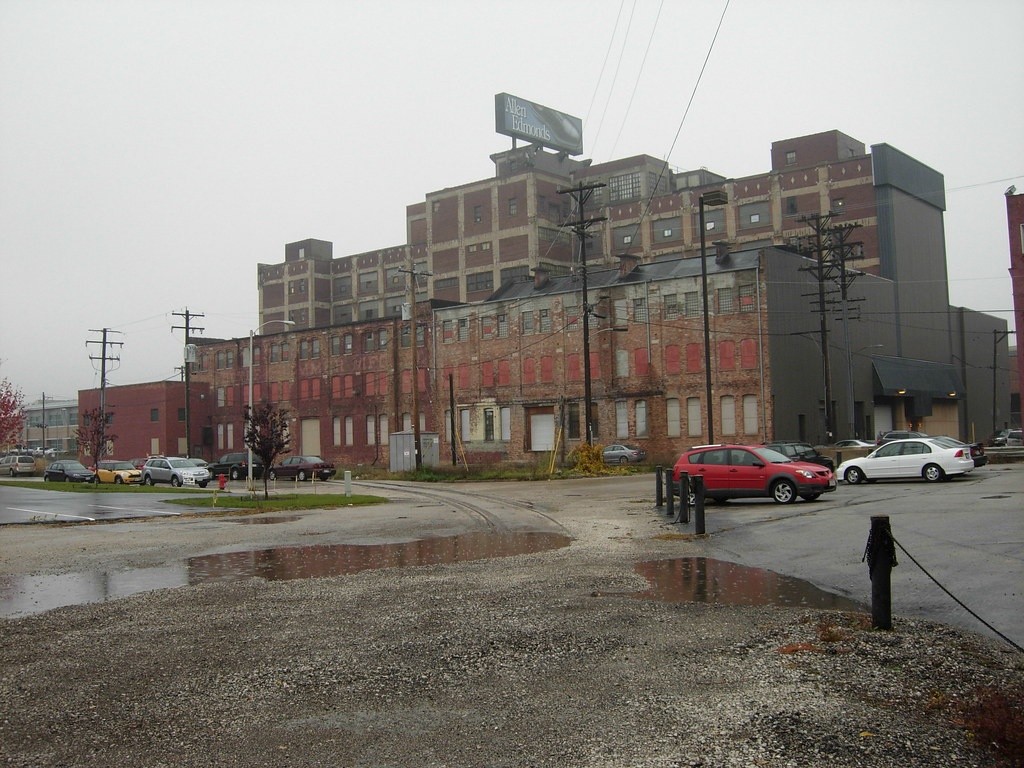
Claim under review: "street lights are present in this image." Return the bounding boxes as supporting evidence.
[246,318,297,490]
[583,326,629,447]
[698,188,729,444]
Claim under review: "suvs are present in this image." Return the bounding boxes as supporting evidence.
[672,443,838,508]
[206,452,264,481]
[0,456,37,477]
[762,439,834,474]
[141,457,211,488]
[876,429,929,445]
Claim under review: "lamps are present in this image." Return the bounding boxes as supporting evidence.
[898,390,906,394]
[948,392,957,397]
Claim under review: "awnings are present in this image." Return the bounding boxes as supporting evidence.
[872,356,967,401]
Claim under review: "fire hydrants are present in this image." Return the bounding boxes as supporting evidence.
[218,474,228,490]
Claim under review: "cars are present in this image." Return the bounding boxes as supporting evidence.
[43,460,96,483]
[10,446,69,457]
[269,456,336,481]
[931,435,987,478]
[993,428,1024,446]
[128,454,209,471]
[93,461,142,484]
[836,437,975,485]
[833,439,876,447]
[602,444,647,465]
